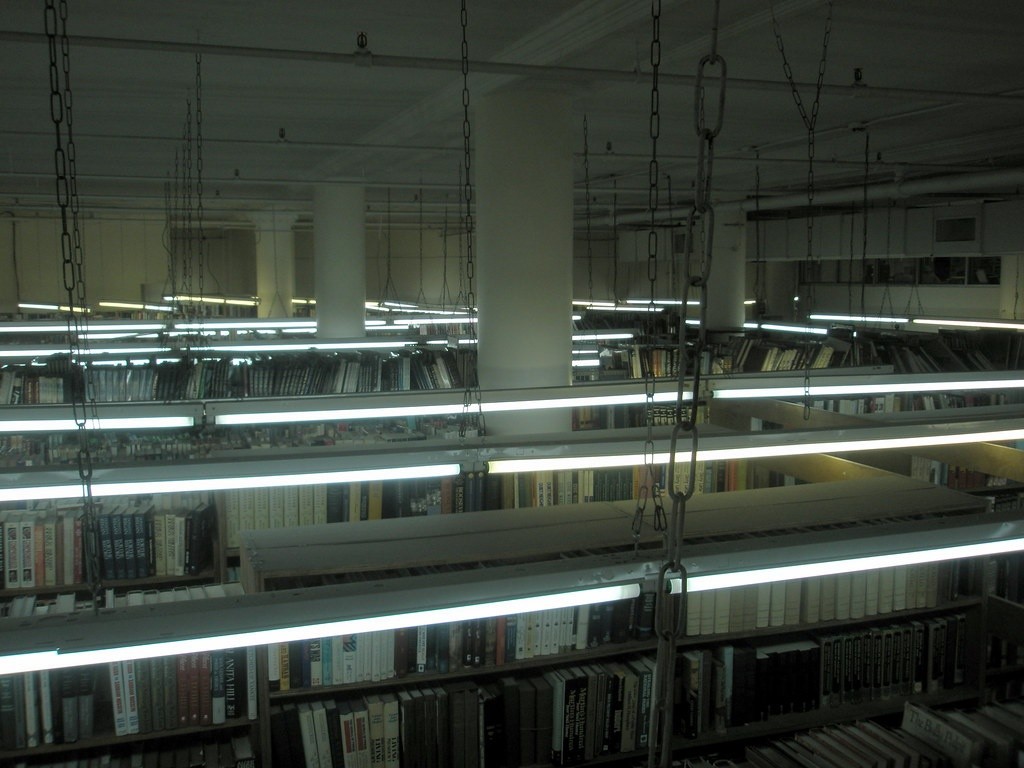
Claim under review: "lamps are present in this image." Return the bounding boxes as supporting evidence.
[480,429,1023,474]
[0,578,642,675]
[806,315,911,322]
[912,319,1023,329]
[704,380,1024,399]
[0,417,197,431]
[204,391,694,426]
[0,461,462,503]
[571,298,702,307]
[667,538,1024,594]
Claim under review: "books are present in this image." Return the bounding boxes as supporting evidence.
[0,332,1024,768]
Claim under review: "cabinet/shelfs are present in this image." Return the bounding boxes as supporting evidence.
[0,305,1024,767]
